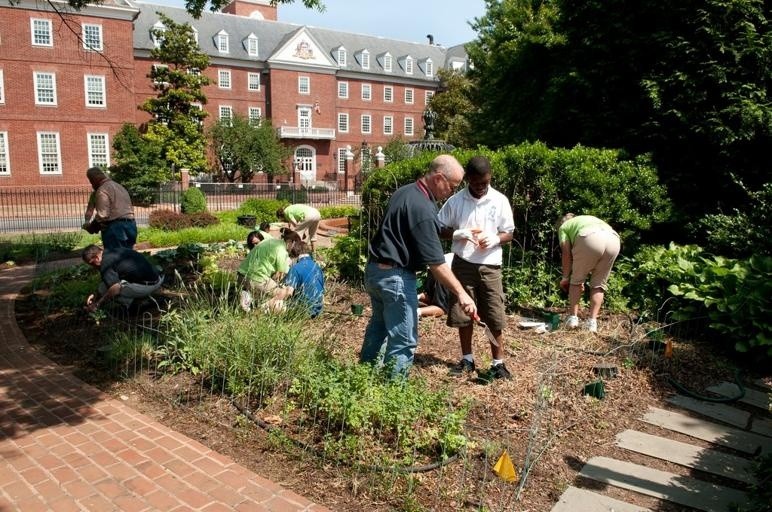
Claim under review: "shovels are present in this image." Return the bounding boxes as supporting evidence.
[470,311,499,348]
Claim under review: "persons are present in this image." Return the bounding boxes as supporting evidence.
[82,244,163,313]
[432,155,516,382]
[246,231,292,282]
[277,203,321,262]
[359,154,466,384]
[281,228,301,259]
[257,241,327,318]
[83,167,136,248]
[258,222,274,239]
[152,243,203,287]
[556,213,622,331]
[235,228,301,313]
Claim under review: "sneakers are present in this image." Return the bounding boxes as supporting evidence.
[455,359,475,373]
[489,364,509,378]
[238,290,254,313]
[564,315,578,329]
[586,318,598,333]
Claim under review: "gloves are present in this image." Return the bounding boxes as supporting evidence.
[480,235,500,250]
[452,229,481,246]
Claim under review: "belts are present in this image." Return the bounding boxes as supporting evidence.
[112,218,135,222]
[368,256,401,268]
[141,273,164,285]
